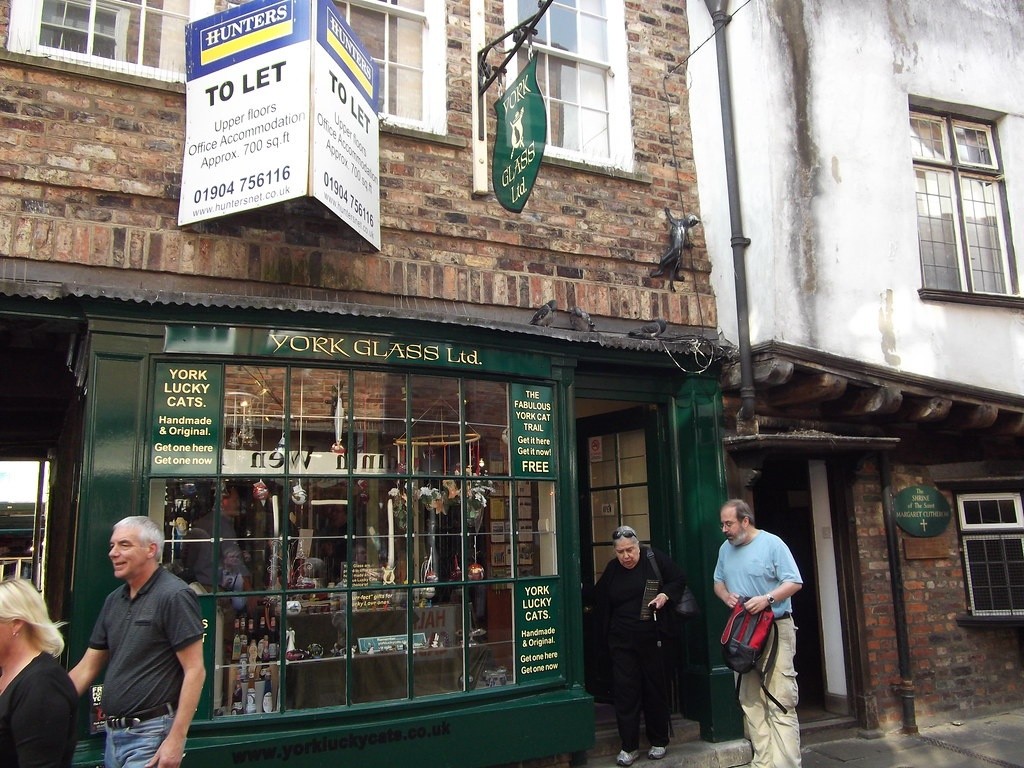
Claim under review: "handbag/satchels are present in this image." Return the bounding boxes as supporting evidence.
[647,549,700,623]
[720,596,788,714]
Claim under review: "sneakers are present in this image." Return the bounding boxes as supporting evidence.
[648,746,667,759]
[616,750,641,766]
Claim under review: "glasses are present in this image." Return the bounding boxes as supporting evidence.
[612,530,637,540]
[720,518,738,530]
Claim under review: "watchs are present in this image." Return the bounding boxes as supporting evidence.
[767,594,774,604]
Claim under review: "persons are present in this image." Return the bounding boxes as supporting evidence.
[69,516,206,768]
[0,579,81,768]
[587,526,685,767]
[187,488,257,715]
[713,499,803,768]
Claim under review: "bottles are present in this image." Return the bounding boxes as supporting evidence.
[245,673,256,715]
[262,671,274,713]
[231,669,244,715]
[229,618,279,682]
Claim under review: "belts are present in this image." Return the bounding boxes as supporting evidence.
[773,611,792,620]
[107,702,177,728]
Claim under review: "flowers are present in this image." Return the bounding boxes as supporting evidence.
[390,467,497,531]
